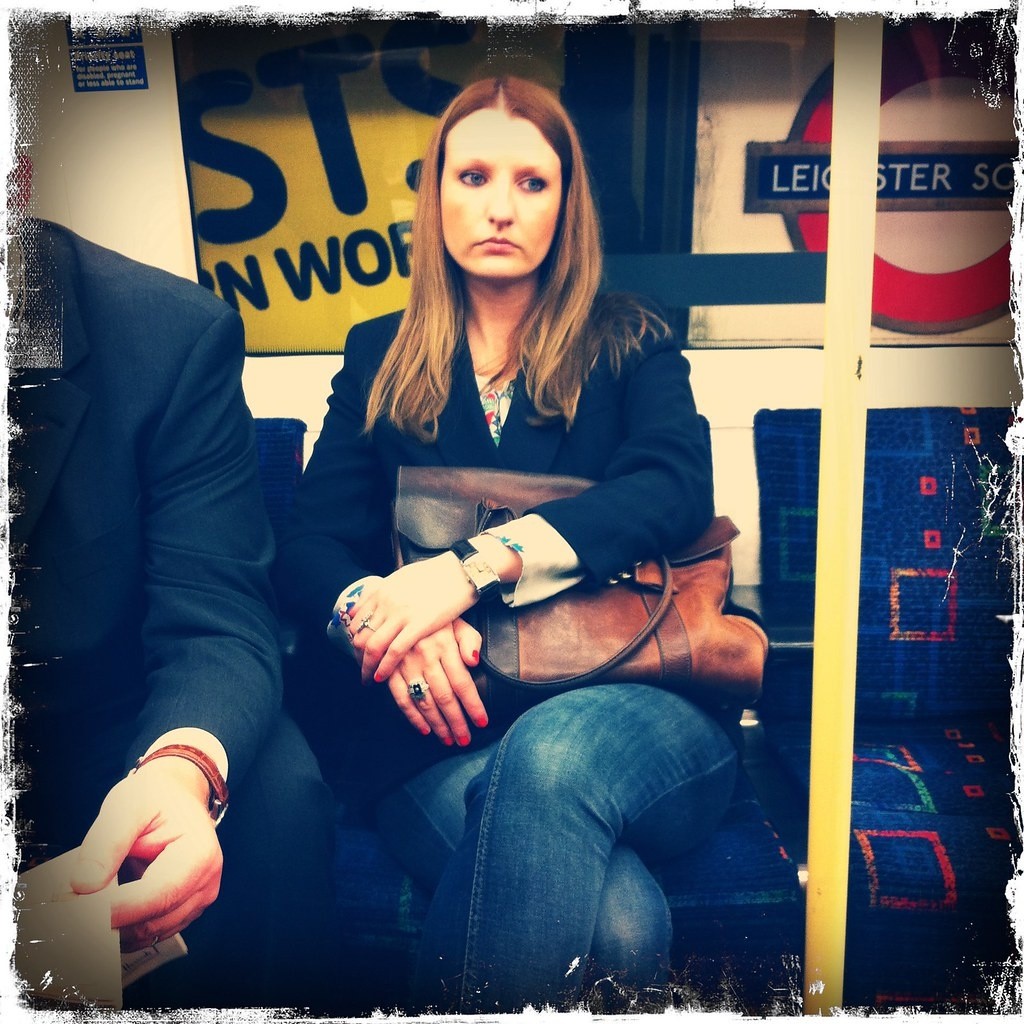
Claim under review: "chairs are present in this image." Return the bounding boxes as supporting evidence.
[753,404,1024,914]
[657,412,797,912]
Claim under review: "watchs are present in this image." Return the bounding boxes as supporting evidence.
[131,744,228,826]
[450,540,502,603]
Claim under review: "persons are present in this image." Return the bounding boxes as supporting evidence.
[12,216,318,1007]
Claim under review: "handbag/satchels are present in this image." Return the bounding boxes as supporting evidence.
[390,465,770,715]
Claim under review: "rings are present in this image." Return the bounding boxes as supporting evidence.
[410,682,428,699]
[280,75,743,1007]
[357,614,376,633]
